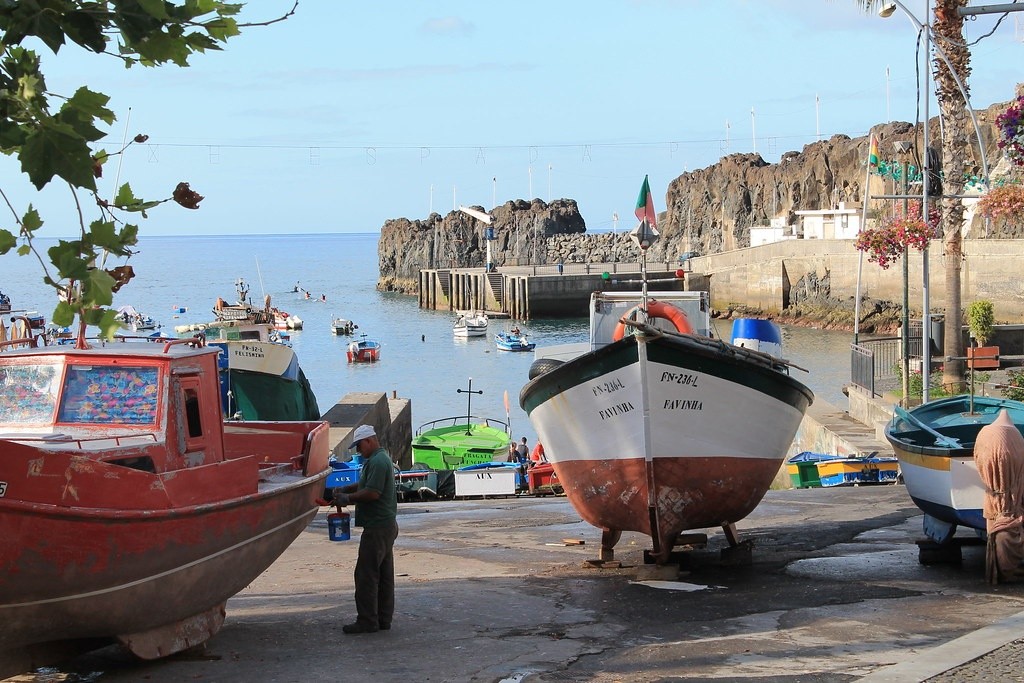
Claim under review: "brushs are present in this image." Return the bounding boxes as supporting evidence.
[315,498,348,507]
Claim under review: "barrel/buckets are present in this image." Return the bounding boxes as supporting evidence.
[325,504,352,541]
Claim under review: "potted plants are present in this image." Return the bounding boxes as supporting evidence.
[966,300,999,368]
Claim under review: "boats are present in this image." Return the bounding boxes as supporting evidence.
[346,332,382,362]
[0,310,331,661]
[42,257,304,350]
[519,210,815,555]
[23,310,46,329]
[884,335,1024,548]
[494,322,537,352]
[331,317,359,336]
[452,290,490,337]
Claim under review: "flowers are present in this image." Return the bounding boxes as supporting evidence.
[852,83,1024,270]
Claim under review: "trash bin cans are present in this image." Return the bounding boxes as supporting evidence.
[929,314,945,356]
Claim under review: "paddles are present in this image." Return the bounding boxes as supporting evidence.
[504,391,511,427]
[894,403,963,448]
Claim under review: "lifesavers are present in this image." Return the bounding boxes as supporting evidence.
[528,359,566,380]
[613,301,694,341]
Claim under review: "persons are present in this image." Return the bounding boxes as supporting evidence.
[121,310,129,323]
[322,294,326,301]
[48,325,70,334]
[328,424,399,633]
[517,438,530,459]
[293,285,298,292]
[490,257,495,272]
[511,326,520,335]
[305,291,310,299]
[136,313,143,322]
[518,334,528,346]
[527,247,532,265]
[507,442,527,486]
[558,253,563,272]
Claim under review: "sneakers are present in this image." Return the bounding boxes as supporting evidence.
[343,620,380,633]
[379,622,391,630]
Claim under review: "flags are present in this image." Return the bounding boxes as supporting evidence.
[634,175,657,228]
[870,134,879,167]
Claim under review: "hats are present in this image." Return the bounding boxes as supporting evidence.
[347,425,376,449]
